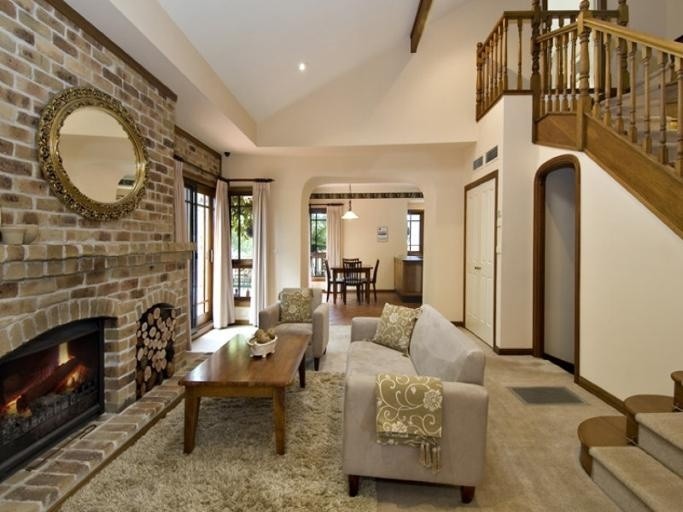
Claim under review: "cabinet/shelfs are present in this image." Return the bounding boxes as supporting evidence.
[463,169,497,348]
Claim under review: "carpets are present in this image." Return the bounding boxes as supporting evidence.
[56,372,381,512]
[505,386,591,408]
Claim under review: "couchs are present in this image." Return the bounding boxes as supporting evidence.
[343,303,488,504]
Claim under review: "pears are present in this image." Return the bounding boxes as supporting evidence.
[254,328,276,344]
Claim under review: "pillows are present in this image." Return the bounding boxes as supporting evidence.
[369,302,422,357]
[278,289,313,323]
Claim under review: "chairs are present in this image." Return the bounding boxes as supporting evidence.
[259,287,329,371]
[323,258,380,306]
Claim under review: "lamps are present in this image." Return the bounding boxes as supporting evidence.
[341,184,359,219]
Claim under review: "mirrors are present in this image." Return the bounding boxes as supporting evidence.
[35,87,153,224]
